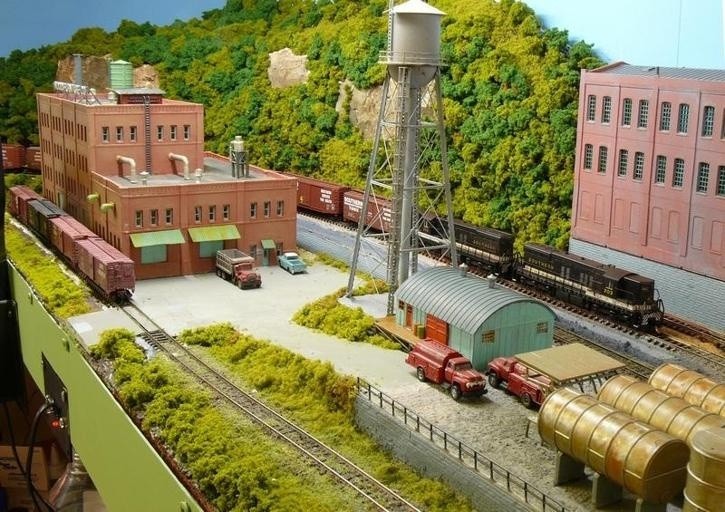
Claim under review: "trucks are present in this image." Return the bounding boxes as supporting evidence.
[485,356,552,409]
[216,249,262,289]
[405,337,488,401]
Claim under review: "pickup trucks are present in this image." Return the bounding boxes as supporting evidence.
[277,252,306,275]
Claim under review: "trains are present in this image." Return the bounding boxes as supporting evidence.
[1,143,41,173]
[8,184,136,306]
[276,171,665,331]
